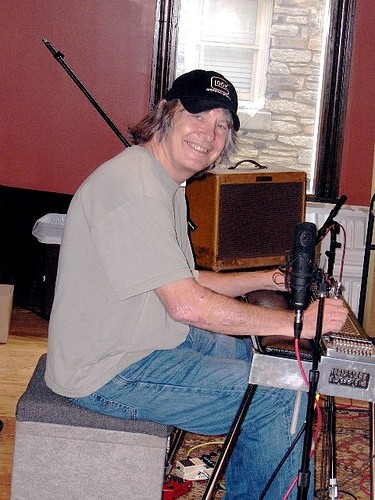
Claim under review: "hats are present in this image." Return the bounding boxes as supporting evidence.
[164,69,241,132]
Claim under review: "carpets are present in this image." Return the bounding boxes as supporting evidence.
[163,394,375,500]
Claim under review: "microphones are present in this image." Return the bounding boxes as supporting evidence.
[290,222,317,340]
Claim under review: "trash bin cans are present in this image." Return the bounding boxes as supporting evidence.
[30,211,68,319]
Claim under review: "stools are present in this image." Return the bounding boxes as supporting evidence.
[11,353,173,500]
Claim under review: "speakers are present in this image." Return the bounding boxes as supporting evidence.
[186,160,307,272]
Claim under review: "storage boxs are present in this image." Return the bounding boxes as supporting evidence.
[0,284,14,344]
[187,159,306,274]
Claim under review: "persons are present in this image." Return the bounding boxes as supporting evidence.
[45,70,349,500]
